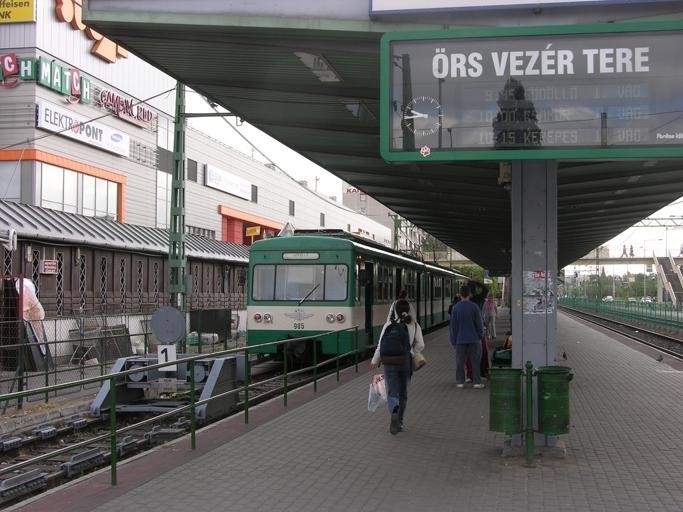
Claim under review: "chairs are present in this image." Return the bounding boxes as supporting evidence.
[68,327,101,366]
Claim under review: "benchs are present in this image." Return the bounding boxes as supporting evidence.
[492,336,512,368]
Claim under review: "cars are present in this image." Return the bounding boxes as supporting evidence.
[624,297,637,306]
[600,295,615,305]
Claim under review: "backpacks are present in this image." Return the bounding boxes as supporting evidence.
[380,323,410,365]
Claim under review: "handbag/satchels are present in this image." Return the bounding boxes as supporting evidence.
[493,349,512,363]
[503,336,512,349]
[410,345,425,371]
[368,374,387,411]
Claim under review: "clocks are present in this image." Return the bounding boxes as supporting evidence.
[404,96,444,136]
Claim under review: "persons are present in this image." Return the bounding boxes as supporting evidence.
[368,299,426,436]
[484,293,498,339]
[447,296,459,315]
[448,285,485,388]
[385,289,416,322]
[448,280,490,383]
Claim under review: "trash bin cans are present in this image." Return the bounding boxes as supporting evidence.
[535,365,574,435]
[488,368,524,434]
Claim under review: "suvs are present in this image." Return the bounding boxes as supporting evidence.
[637,295,653,305]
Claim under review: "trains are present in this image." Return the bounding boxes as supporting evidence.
[246,224,478,375]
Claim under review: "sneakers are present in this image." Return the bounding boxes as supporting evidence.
[457,377,488,388]
[390,406,403,434]
